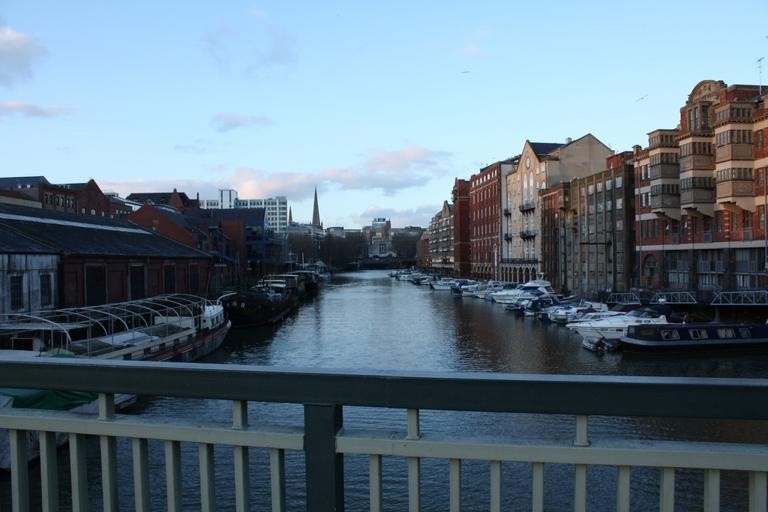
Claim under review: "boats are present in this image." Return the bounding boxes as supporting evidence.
[1,290,232,473]
[215,261,328,328]
[618,320,766,354]
[583,335,614,355]
[385,267,628,326]
[565,305,669,341]
